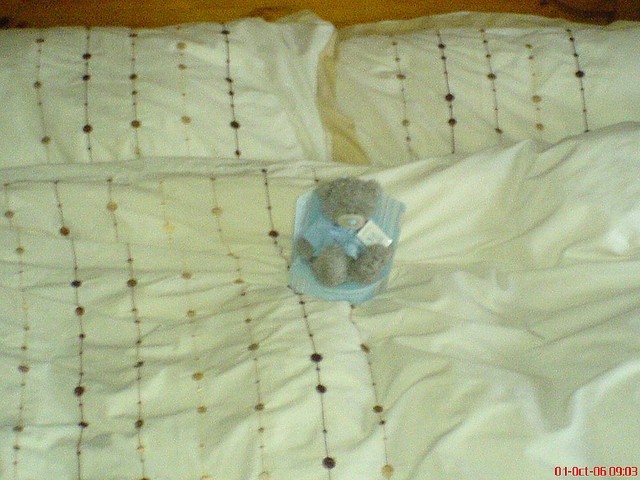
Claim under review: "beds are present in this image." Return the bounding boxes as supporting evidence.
[1,1,638,480]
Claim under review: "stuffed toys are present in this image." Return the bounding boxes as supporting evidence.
[299,177,393,288]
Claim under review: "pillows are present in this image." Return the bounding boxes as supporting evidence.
[1,9,338,165]
[315,8,640,167]
